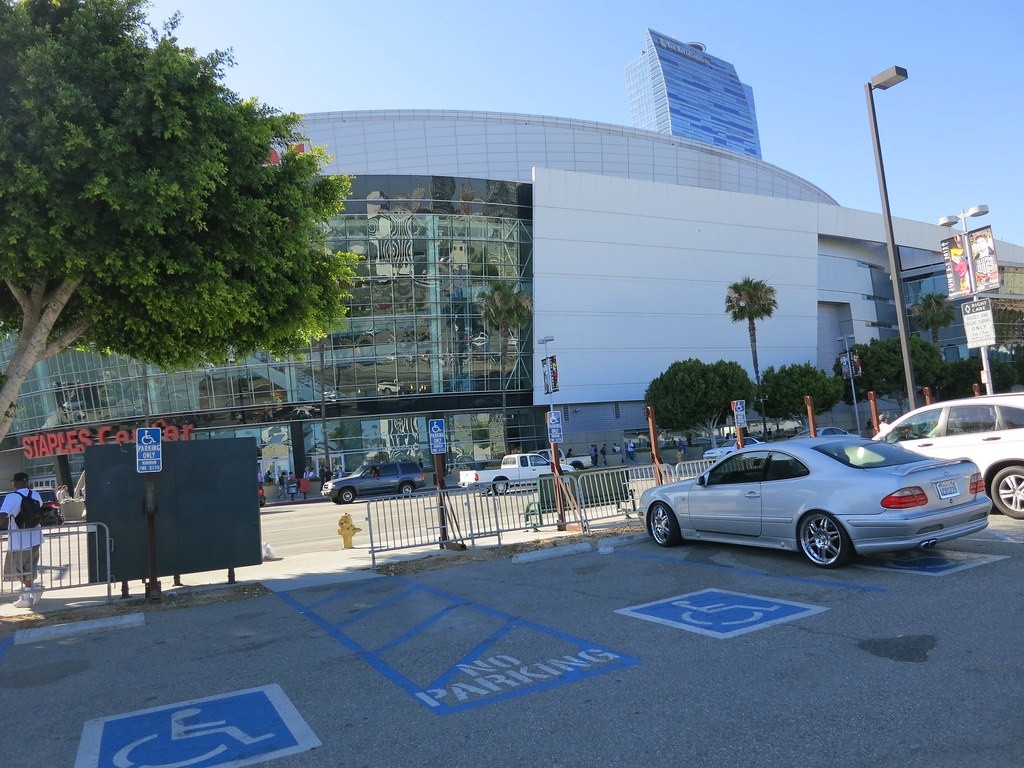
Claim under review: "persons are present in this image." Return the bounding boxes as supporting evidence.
[418,458,423,467]
[369,425,386,451]
[288,471,296,480]
[319,465,332,492]
[879,414,895,441]
[733,432,737,438]
[278,472,287,499]
[57,485,69,514]
[370,467,379,476]
[335,467,345,478]
[670,436,676,444]
[841,355,848,366]
[678,437,686,455]
[566,448,572,458]
[589,439,635,467]
[975,236,994,259]
[647,436,651,447]
[0,473,43,608]
[726,432,730,439]
[867,418,872,430]
[759,428,772,437]
[744,430,751,436]
[81,485,86,500]
[264,470,273,483]
[304,466,319,479]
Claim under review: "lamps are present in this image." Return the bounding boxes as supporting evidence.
[574,408,580,412]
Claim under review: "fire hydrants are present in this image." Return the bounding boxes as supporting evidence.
[338,513,362,549]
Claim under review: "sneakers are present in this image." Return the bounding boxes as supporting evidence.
[32,586,45,604]
[13,594,31,609]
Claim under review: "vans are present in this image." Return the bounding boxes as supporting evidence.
[0,487,65,533]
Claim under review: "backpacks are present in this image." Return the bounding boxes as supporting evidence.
[630,442,636,448]
[600,447,606,455]
[12,490,41,530]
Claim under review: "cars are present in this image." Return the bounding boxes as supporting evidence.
[702,436,767,462]
[871,390,1024,521]
[790,425,860,442]
[636,432,995,570]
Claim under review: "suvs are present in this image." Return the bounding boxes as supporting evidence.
[320,456,428,505]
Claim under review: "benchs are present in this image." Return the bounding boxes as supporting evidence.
[523,465,637,534]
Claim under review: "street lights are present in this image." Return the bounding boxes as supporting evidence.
[864,65,922,433]
[835,333,861,437]
[537,335,567,531]
[938,203,995,416]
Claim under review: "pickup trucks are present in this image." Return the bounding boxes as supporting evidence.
[457,452,576,496]
[527,447,593,471]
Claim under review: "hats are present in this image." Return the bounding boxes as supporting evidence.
[878,413,889,421]
[10,472,28,482]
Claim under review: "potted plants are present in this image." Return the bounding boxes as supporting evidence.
[451,467,470,482]
[485,462,501,470]
[262,481,279,500]
[59,497,86,521]
